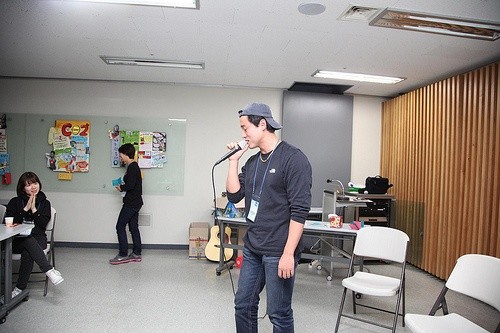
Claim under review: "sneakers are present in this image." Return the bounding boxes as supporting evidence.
[128,252,142,262]
[0,286,22,304]
[109,254,129,264]
[45,267,63,286]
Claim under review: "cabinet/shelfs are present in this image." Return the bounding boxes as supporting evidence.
[342,195,395,264]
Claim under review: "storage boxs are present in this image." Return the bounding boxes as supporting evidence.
[216,197,246,218]
[189,222,210,260]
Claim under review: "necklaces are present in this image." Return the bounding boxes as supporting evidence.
[259,150,271,163]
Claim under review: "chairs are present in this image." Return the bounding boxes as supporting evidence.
[0,204,57,295]
[334,225,411,333]
[403,253,500,333]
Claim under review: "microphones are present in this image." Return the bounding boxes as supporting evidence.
[327,179,345,197]
[214,140,247,166]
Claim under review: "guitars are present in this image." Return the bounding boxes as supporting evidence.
[204,225,234,262]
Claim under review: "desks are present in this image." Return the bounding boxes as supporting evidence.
[214,216,371,299]
[0,224,34,324]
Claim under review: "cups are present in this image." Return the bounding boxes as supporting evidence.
[5,217,14,227]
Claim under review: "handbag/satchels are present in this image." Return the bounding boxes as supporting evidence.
[366,175,389,194]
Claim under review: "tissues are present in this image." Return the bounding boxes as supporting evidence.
[328,213,343,229]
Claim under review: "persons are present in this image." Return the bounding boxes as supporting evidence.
[109,143,143,265]
[226,102,313,333]
[0,172,65,305]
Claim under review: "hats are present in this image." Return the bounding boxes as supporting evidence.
[239,103,282,130]
